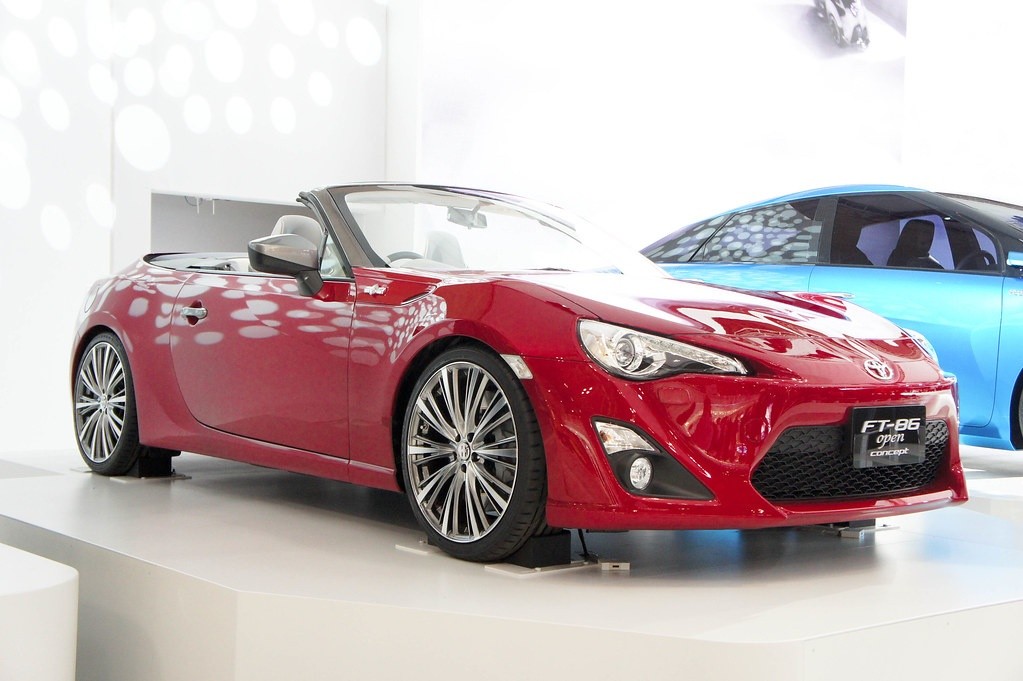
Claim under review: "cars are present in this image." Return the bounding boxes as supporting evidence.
[641,184,1019,450]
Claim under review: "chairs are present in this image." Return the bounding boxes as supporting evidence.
[270,214,330,259]
[424,231,465,268]
[886,219,944,269]
[831,214,874,265]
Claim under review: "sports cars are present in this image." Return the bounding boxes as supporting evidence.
[70,184,967,567]
